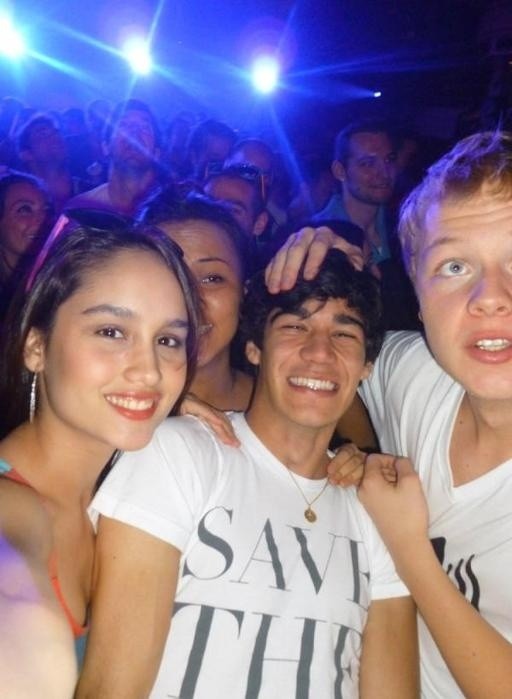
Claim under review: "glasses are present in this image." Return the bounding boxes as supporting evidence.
[203,159,265,200]
[24,207,184,305]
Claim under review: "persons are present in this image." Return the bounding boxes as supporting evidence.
[265,134,512,699]
[0,220,241,699]
[1,86,512,490]
[73,248,421,699]
